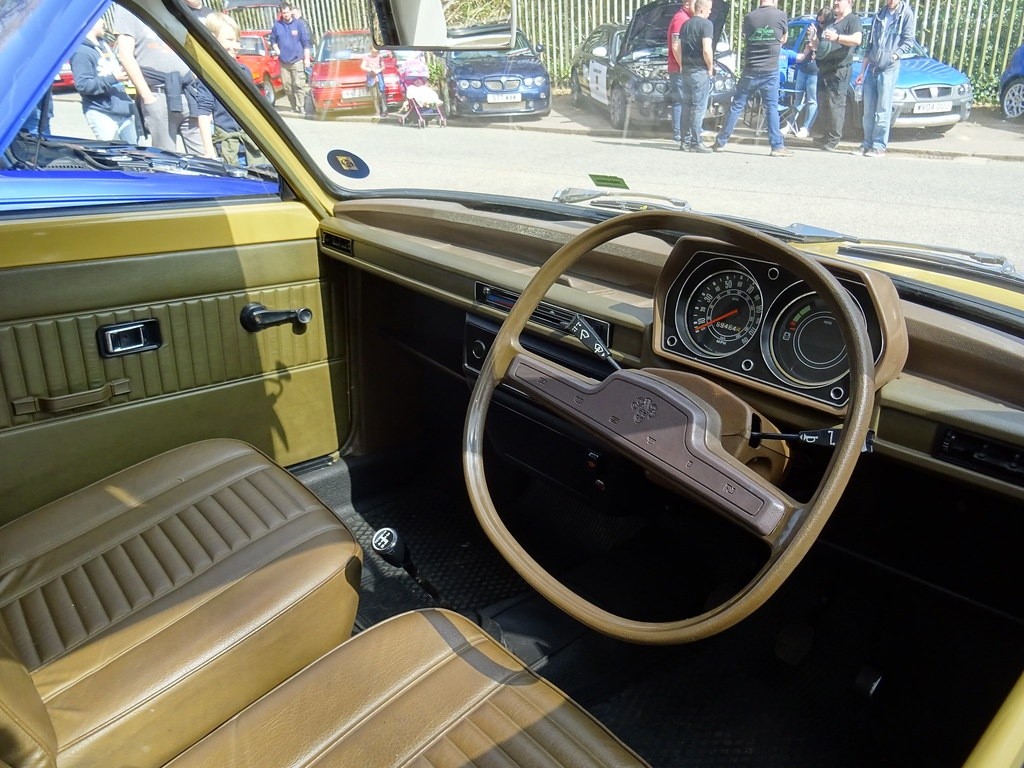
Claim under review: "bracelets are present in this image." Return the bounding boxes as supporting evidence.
[836,34,840,43]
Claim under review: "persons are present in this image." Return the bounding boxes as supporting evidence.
[780,7,835,137]
[850,0,915,156]
[393,50,443,125]
[668,0,698,142]
[764,44,810,130]
[679,0,713,153]
[113,0,216,157]
[270,2,312,116]
[710,0,795,157]
[196,13,267,167]
[360,44,389,117]
[70,17,139,147]
[807,0,862,149]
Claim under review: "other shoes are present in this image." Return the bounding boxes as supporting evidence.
[299,107,306,114]
[796,127,809,139]
[824,140,839,150]
[373,113,380,118]
[381,112,389,119]
[780,126,793,135]
[851,145,867,156]
[865,148,887,157]
[813,137,829,145]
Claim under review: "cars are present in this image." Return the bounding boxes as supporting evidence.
[234,30,286,106]
[570,0,743,132]
[51,36,138,96]
[0,0,1024,768]
[775,11,973,137]
[310,30,407,118]
[439,22,552,120]
[998,39,1024,122]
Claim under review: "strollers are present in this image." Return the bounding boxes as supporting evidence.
[394,59,448,128]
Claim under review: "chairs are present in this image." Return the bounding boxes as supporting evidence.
[743,88,807,137]
[0,435,651,768]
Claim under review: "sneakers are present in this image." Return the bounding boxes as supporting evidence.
[770,146,795,157]
[709,143,723,152]
[680,142,690,151]
[689,143,712,153]
